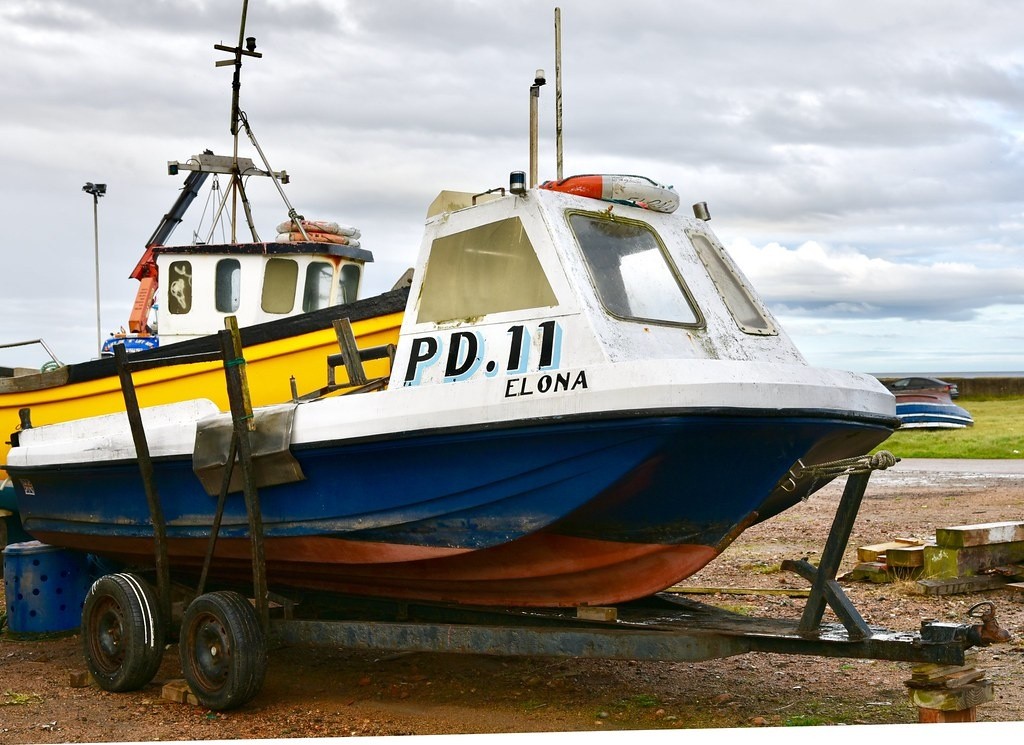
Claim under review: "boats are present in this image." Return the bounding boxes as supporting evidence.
[891,388,975,430]
[0,0,903,616]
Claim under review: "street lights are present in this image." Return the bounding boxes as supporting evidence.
[80,180,108,354]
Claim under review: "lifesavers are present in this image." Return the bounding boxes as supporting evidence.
[287,233,362,247]
[544,173,682,213]
[276,219,363,240]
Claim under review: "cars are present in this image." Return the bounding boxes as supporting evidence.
[887,376,959,399]
